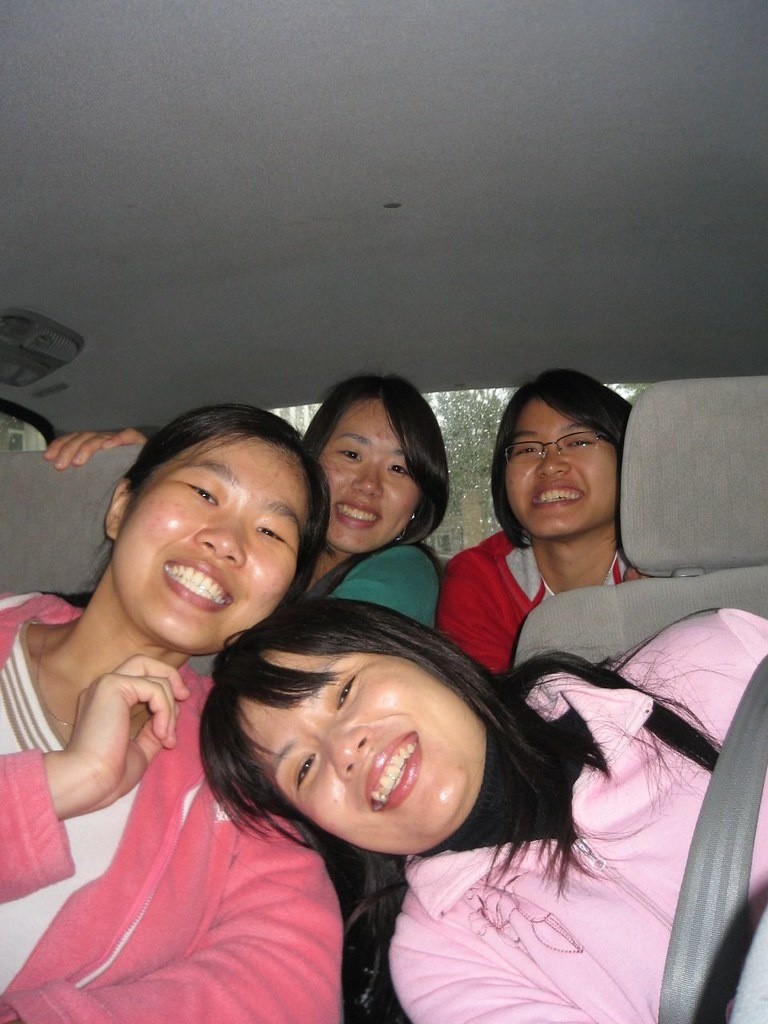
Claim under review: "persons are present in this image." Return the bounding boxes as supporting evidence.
[38,374,450,632]
[196,597,766,1024]
[436,369,654,691]
[0,403,348,1024]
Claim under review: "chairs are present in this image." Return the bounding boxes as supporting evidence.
[365,374,768,895]
[0,444,320,855]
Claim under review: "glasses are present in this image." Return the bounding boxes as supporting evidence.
[504,430,606,466]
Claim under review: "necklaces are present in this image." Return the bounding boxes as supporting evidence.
[35,625,148,728]
